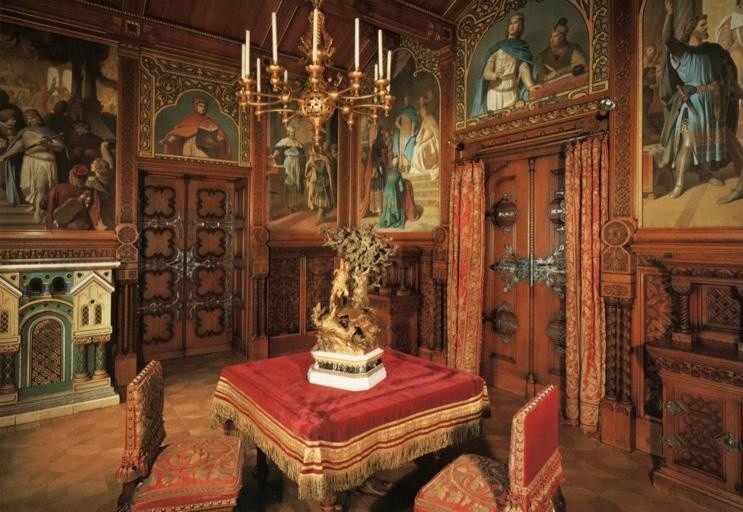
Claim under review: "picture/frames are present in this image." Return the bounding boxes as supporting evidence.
[634,0,743,230]
[454,0,610,133]
[258,86,344,238]
[0,7,125,233]
[356,38,450,240]
[138,52,252,167]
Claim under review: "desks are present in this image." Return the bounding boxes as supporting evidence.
[212,347,490,512]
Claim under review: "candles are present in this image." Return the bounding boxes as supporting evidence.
[386,49,391,93]
[284,69,288,83]
[256,58,261,98]
[245,30,250,77]
[377,29,383,79]
[241,43,245,80]
[271,11,277,65]
[355,17,359,70]
[373,64,377,102]
[312,8,317,63]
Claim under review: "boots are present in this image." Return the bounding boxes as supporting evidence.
[698,161,724,186]
[670,145,693,198]
[316,208,325,225]
[716,169,743,204]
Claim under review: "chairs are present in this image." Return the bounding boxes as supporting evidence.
[114,359,245,512]
[414,382,567,512]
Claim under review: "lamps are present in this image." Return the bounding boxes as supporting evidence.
[234,0,397,158]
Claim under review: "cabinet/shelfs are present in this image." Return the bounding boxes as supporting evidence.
[368,294,419,354]
[662,374,743,498]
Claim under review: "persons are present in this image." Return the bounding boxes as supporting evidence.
[0,109,18,148]
[662,0,738,199]
[306,143,335,225]
[377,157,404,228]
[383,128,393,152]
[84,142,115,201]
[361,124,388,219]
[535,17,586,82]
[158,97,232,162]
[643,45,665,145]
[53,102,92,182]
[483,13,542,112]
[409,105,439,174]
[46,164,108,232]
[418,95,427,108]
[427,89,435,102]
[0,114,64,225]
[266,124,304,214]
[395,96,419,173]
